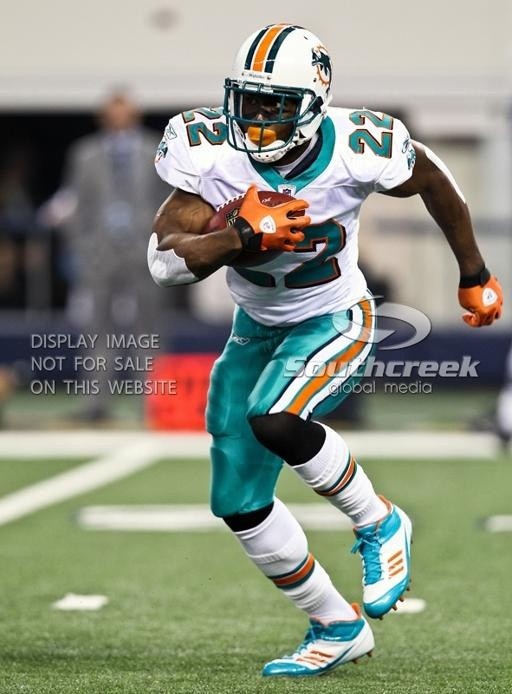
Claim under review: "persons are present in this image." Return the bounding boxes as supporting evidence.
[141,16,505,681]
[57,84,176,432]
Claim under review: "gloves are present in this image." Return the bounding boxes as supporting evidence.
[233,183,313,256]
[457,264,506,330]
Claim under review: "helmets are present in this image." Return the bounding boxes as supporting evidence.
[221,21,334,164]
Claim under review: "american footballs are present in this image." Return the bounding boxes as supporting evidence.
[203,191,305,266]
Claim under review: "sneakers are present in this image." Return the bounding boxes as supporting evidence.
[261,601,377,679]
[351,494,414,620]
